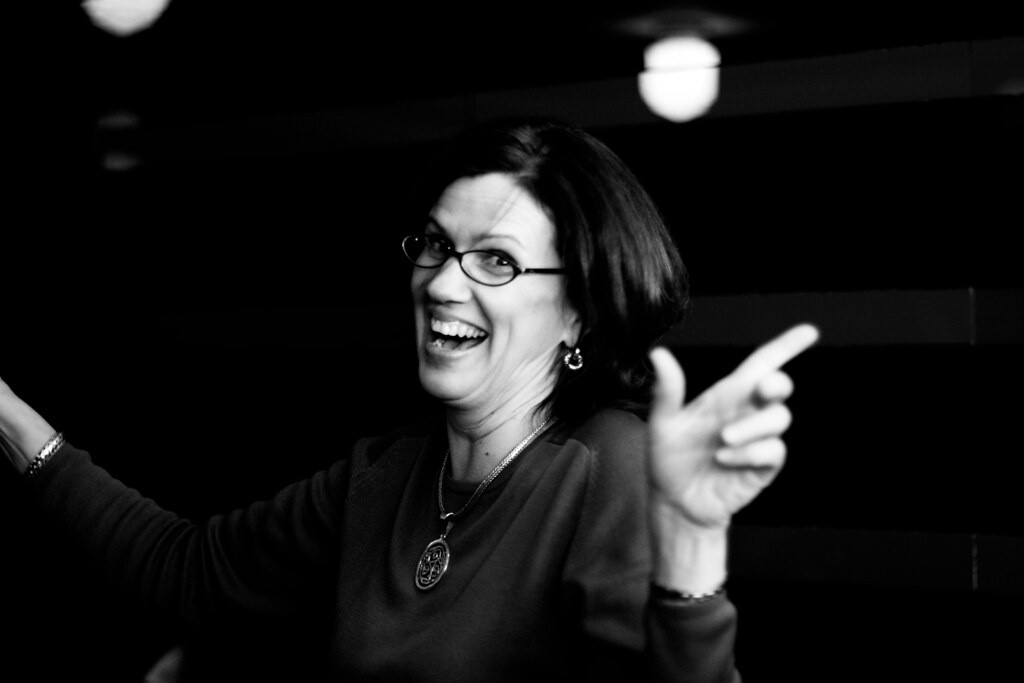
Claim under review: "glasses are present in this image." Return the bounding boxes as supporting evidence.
[401,233,568,287]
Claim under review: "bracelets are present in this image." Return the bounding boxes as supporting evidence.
[0,117,825,683]
[19,429,67,480]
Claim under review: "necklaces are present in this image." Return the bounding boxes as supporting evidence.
[413,417,561,592]
